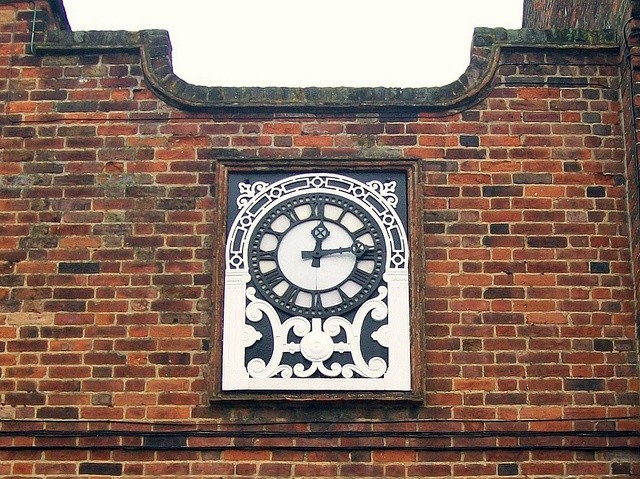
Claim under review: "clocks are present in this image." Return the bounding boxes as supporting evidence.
[208,158,426,402]
